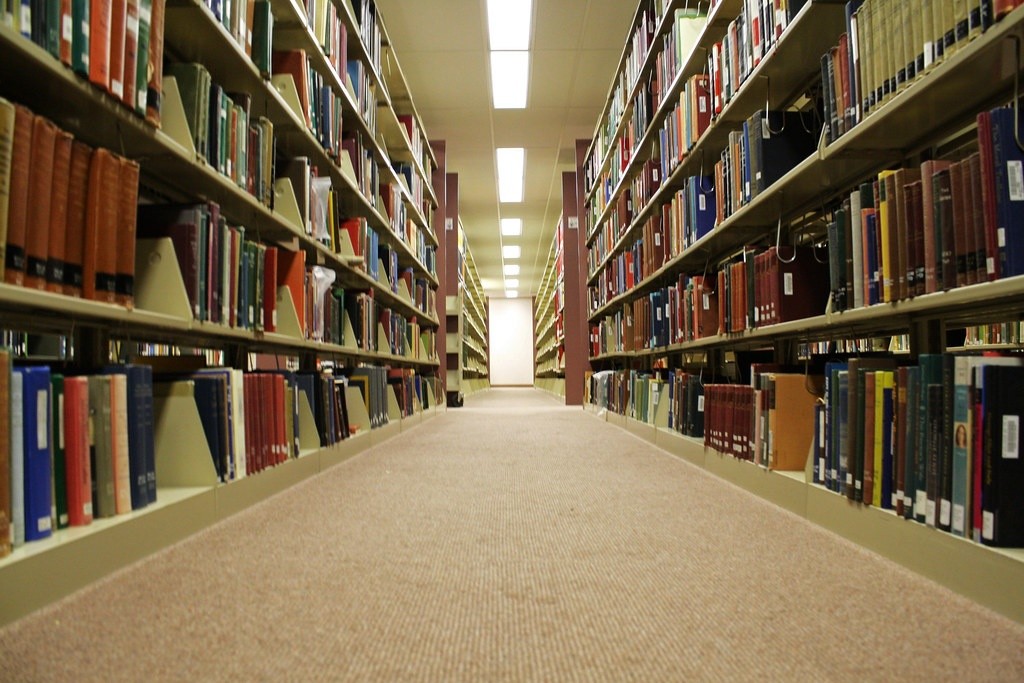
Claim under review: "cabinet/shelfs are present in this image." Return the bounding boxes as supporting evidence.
[446,172,490,407]
[1,0,446,627]
[575,0,1024,626]
[532,171,580,405]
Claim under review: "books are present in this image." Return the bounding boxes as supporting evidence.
[552,217,567,371]
[0,0,442,564]
[455,223,470,370]
[582,0,1024,562]
